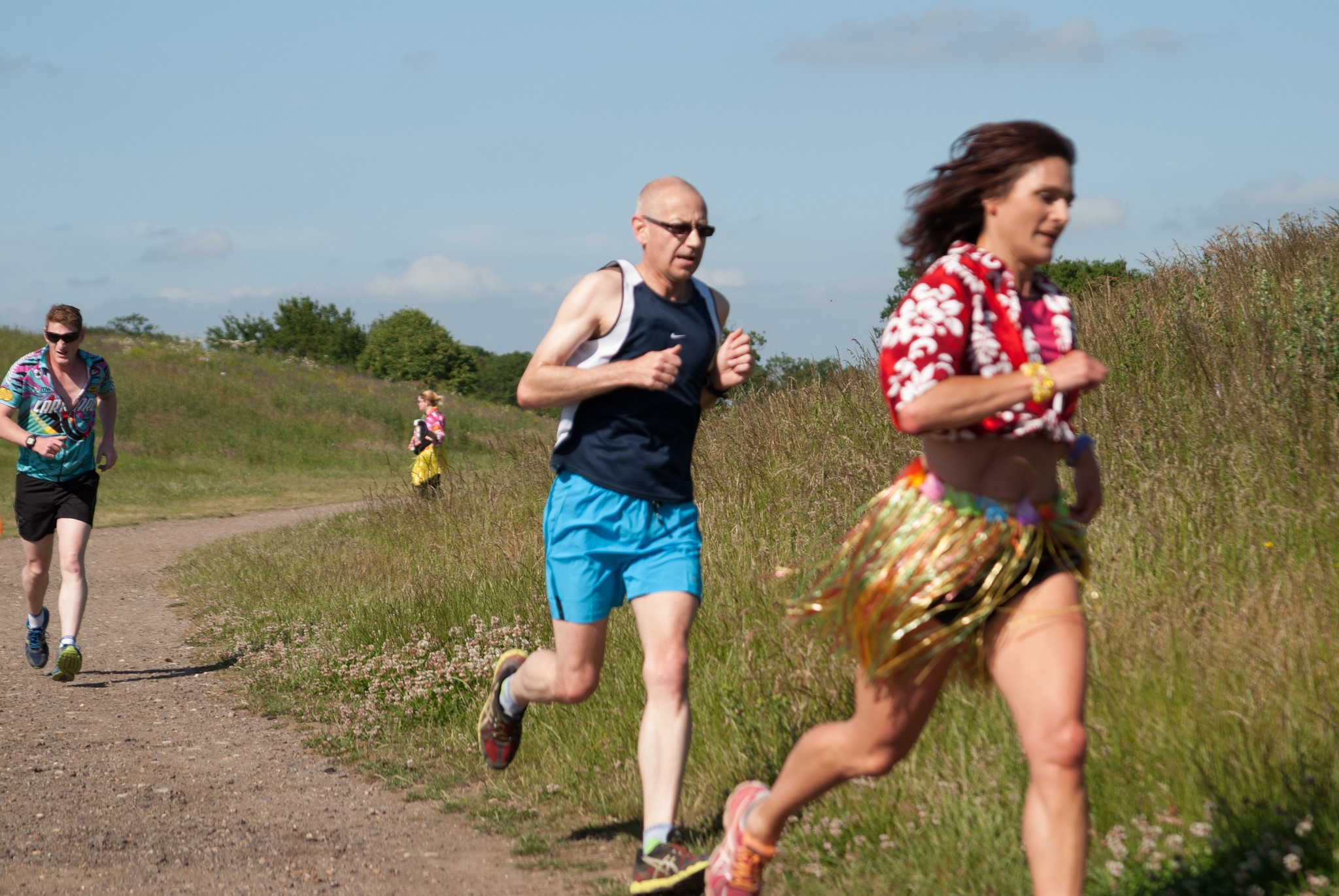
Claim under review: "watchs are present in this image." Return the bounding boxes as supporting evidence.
[25,433,38,451]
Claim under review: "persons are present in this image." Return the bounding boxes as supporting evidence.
[406,390,447,499]
[476,174,754,895]
[704,120,1105,896]
[0,304,117,679]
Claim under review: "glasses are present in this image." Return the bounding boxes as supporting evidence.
[417,399,427,403]
[45,330,82,343]
[642,213,715,237]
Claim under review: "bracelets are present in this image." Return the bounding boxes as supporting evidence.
[1019,363,1055,403]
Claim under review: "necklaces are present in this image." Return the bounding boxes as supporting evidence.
[1018,291,1044,301]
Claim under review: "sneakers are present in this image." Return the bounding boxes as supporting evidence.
[477,649,530,770]
[705,781,779,896]
[53,642,82,682]
[26,604,52,671]
[630,829,710,896]
